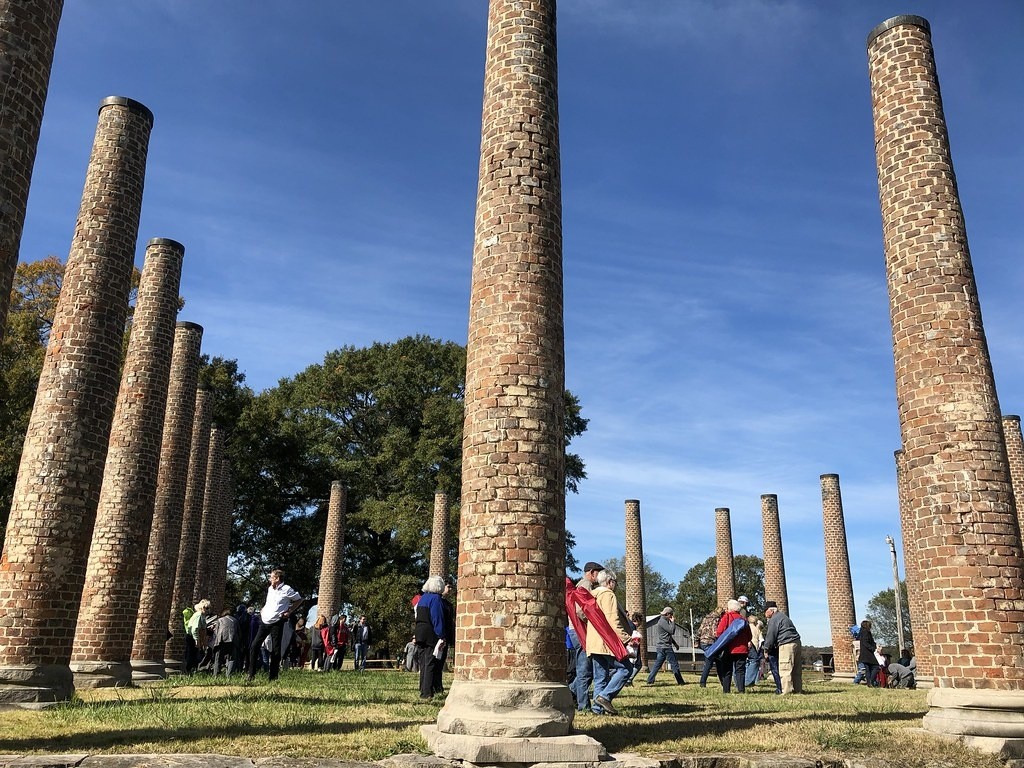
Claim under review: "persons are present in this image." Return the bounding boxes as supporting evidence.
[695,596,803,695]
[850,621,916,690]
[247,569,302,681]
[646,607,687,686]
[403,575,455,699]
[310,615,372,674]
[183,599,306,680]
[568,561,643,715]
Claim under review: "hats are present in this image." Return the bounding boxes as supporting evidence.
[411,634,416,638]
[660,607,673,615]
[738,596,749,602]
[584,562,605,572]
[764,600,777,610]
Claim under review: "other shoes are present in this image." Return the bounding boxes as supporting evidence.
[625,681,634,687]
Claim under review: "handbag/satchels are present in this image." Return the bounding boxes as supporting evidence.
[199,627,216,647]
[703,617,749,661]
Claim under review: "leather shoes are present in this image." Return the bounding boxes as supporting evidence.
[595,695,619,715]
